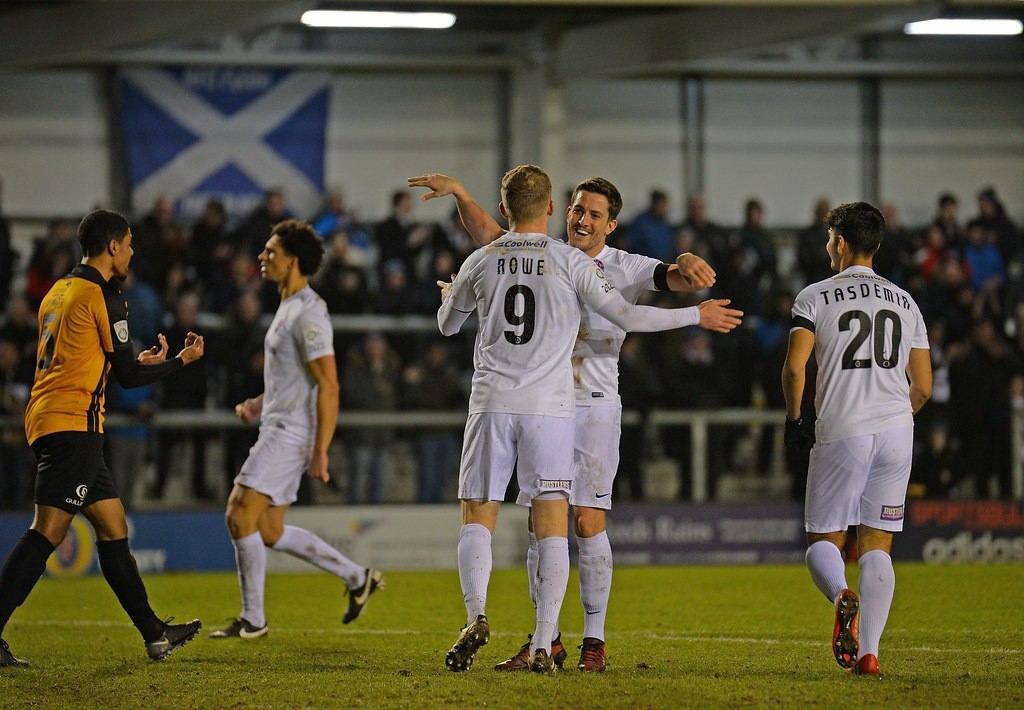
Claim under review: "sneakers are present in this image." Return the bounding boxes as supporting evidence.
[147,616,200,660]
[523,645,560,676]
[0,640,30,669]
[442,615,489,669]
[577,635,608,672]
[496,628,567,668]
[851,653,885,678]
[340,567,383,625]
[833,590,861,667]
[206,615,269,640]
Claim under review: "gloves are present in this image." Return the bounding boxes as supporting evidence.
[782,416,814,455]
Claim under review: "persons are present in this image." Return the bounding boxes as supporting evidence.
[406,165,717,673]
[206,215,384,638]
[435,163,745,677]
[0,163,1024,507]
[781,201,932,676]
[0,206,206,669]
